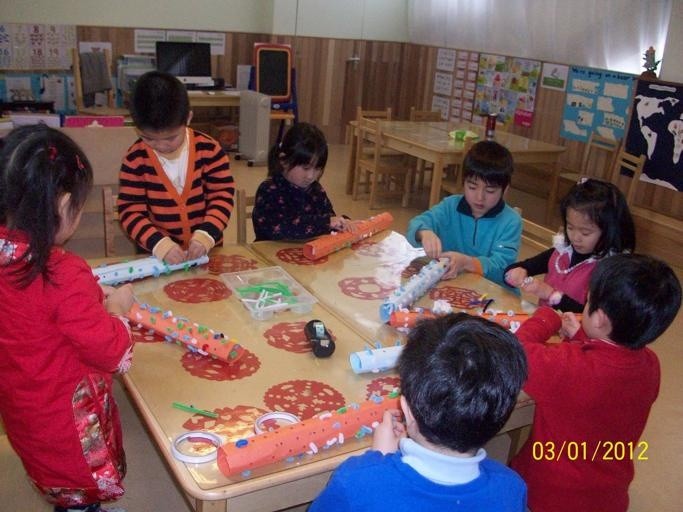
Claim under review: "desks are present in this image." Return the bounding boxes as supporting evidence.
[345,118,567,228]
[84,240,422,512]
[246,224,599,463]
[187,81,240,108]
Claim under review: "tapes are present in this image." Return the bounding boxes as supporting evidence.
[171,431,223,464]
[255,411,301,434]
[313,321,324,339]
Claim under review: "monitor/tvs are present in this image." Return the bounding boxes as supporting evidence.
[155,41,213,83]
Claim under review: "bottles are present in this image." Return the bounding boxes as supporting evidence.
[485,113,497,137]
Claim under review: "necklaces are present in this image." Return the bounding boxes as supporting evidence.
[553,241,600,274]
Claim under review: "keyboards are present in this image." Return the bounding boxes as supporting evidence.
[187,90,203,96]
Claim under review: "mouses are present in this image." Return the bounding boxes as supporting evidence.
[207,91,216,95]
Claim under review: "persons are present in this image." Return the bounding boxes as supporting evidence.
[505,177,637,313]
[306,312,527,511]
[250,120,359,239]
[117,72,235,264]
[402,138,523,296]
[1,124,136,512]
[508,252,681,510]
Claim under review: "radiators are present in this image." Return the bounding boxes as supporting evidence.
[234,90,274,167]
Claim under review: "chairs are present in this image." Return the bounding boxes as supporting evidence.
[72,47,134,122]
[550,131,621,194]
[101,184,227,255]
[508,209,564,263]
[411,107,460,190]
[356,107,410,201]
[598,152,647,206]
[442,135,474,198]
[235,189,255,243]
[352,119,411,208]
